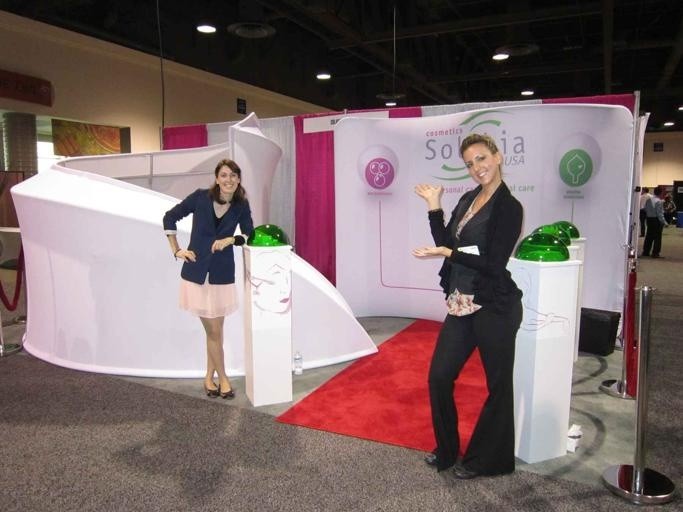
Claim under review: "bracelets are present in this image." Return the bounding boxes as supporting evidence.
[172,248,181,258]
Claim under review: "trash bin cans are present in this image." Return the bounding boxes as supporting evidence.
[677,211,683,228]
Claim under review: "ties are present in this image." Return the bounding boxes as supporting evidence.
[219,384,235,399]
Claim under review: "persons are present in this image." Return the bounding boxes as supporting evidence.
[662,193,677,223]
[409,130,525,477]
[160,156,254,399]
[638,185,669,259]
[639,187,652,237]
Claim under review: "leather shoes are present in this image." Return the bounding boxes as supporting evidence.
[453,466,480,480]
[203,380,220,398]
[422,451,438,467]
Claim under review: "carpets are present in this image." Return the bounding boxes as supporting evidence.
[272,317,488,459]
